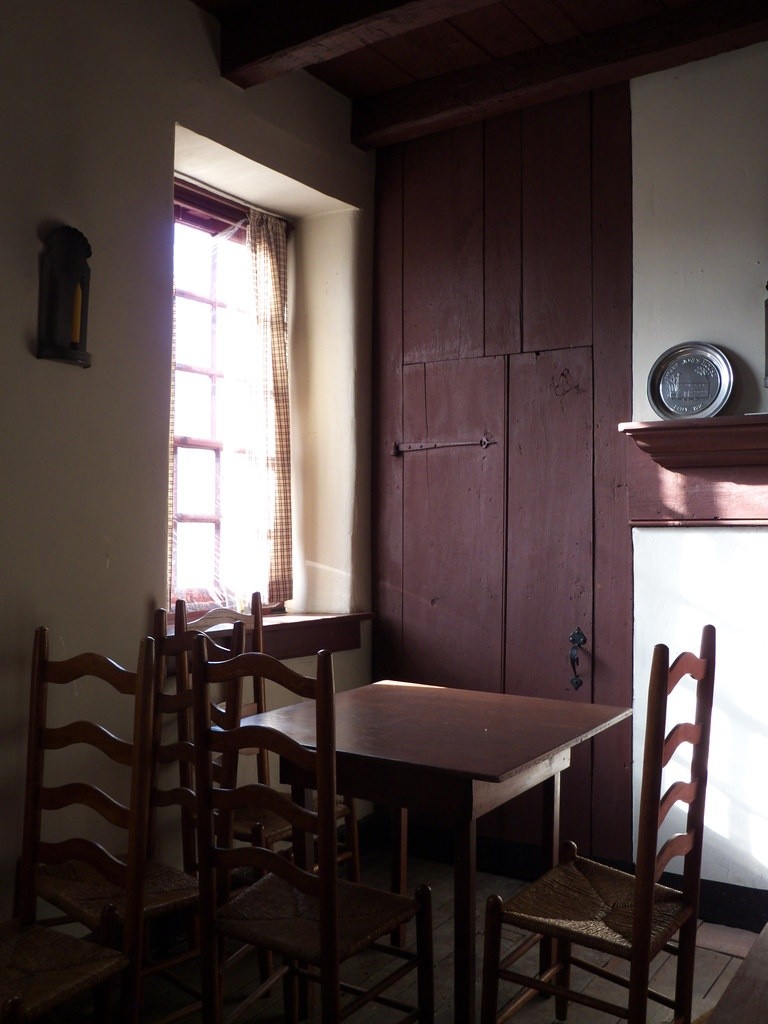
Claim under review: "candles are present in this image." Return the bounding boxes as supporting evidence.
[71,281,81,344]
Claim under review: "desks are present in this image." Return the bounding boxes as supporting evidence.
[212,678,633,1024]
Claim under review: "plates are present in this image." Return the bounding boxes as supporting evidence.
[646,341,734,421]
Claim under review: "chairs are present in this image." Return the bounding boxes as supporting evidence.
[480,624,717,1024]
[0,591,434,1024]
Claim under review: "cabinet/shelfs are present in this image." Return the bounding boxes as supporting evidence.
[374,86,633,878]
